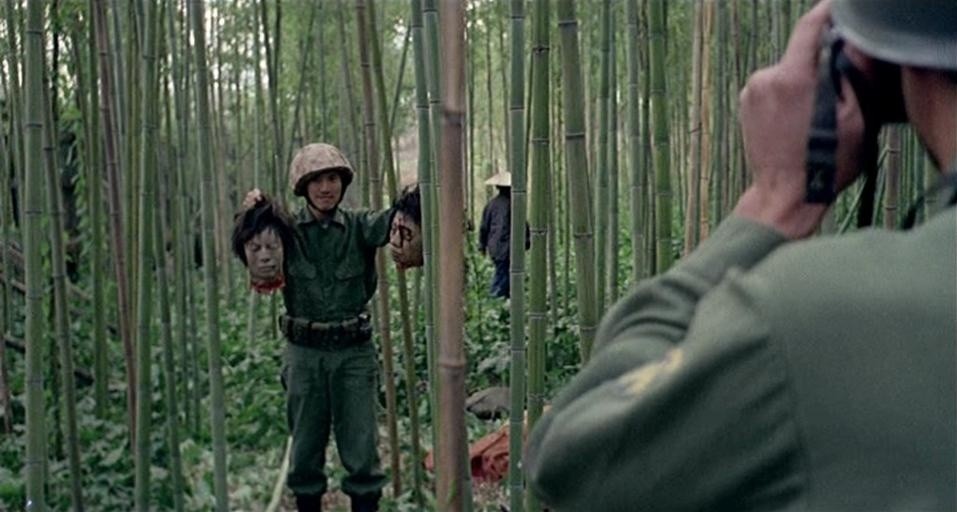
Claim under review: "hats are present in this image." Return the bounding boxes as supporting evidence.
[485,171,511,186]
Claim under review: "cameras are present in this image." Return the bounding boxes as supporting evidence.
[829,47,910,124]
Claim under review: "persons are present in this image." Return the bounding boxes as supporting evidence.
[388,181,423,271]
[242,142,404,512]
[476,172,531,301]
[523,0,957,512]
[229,193,302,296]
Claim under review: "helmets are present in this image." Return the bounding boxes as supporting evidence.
[289,142,354,196]
[830,1,957,69]
